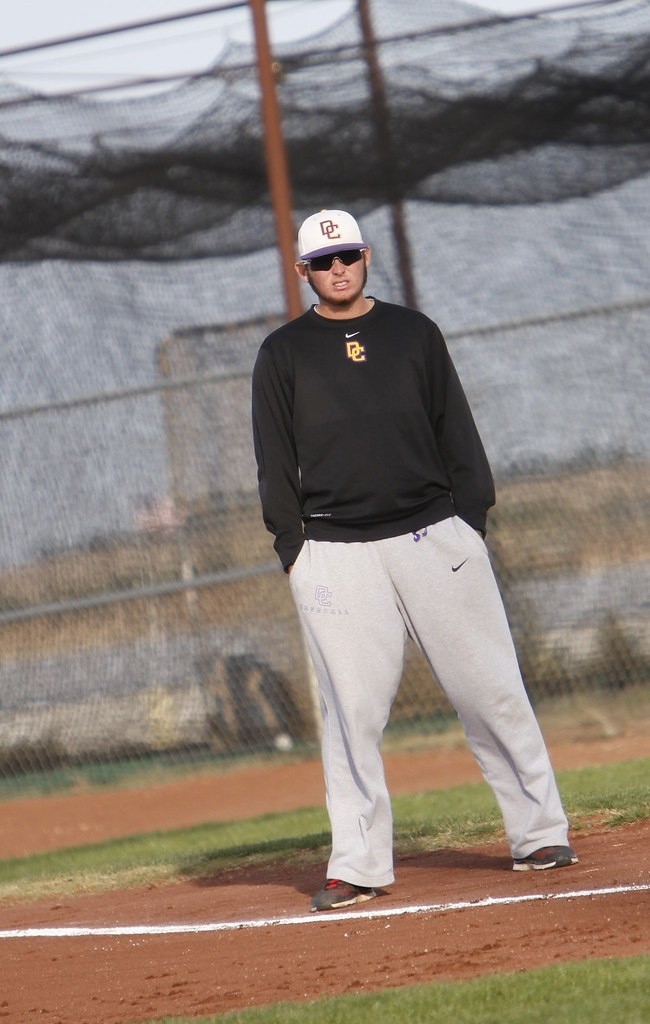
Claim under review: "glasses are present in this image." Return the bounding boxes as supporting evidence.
[301,248,364,270]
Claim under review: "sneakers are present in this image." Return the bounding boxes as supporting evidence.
[513,846,578,871]
[310,879,377,912]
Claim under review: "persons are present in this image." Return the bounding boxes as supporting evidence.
[253,209,580,911]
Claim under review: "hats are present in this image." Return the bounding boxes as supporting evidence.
[298,209,368,260]
